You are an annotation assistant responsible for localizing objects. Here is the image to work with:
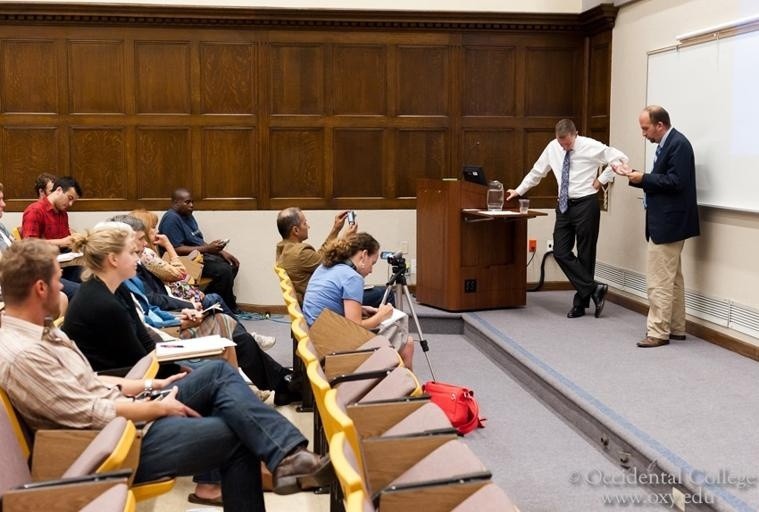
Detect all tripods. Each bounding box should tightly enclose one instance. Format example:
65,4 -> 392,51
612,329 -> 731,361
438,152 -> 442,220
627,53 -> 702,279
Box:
379,267 -> 436,382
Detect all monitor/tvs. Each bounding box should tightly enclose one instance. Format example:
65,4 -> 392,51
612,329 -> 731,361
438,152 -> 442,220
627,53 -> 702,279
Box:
463,165 -> 487,186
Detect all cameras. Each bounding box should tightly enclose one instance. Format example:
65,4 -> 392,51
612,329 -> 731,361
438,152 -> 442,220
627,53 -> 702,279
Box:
380,249 -> 406,267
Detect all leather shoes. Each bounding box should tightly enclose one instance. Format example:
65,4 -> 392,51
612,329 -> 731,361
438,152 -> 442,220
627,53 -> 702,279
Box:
272,446 -> 336,494
637,337 -> 669,347
670,334 -> 686,340
592,283 -> 607,319
568,306 -> 585,318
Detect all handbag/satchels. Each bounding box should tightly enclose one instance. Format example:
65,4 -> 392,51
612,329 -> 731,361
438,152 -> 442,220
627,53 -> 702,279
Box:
170,280 -> 204,300
423,381 -> 473,427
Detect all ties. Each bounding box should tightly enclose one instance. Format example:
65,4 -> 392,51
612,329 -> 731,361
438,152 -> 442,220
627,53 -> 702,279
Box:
558,150 -> 572,213
643,145 -> 660,208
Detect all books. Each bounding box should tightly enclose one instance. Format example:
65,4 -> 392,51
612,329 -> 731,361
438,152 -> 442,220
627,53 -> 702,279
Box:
374,304 -> 407,337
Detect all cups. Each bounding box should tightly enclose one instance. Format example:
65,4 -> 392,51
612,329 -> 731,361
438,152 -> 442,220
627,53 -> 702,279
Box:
519,199 -> 530,213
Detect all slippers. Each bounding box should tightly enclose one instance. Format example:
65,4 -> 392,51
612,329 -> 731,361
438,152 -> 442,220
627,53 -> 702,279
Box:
189,492 -> 223,507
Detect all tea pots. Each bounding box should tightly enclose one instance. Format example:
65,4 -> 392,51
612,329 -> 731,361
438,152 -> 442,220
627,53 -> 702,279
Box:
486,180 -> 505,211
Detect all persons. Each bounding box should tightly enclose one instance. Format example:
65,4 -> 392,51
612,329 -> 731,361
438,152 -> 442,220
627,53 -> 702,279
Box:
273,205 -> 360,294
301,231 -> 414,373
0,171 -> 335,511
505,116 -> 630,319
609,103 -> 702,349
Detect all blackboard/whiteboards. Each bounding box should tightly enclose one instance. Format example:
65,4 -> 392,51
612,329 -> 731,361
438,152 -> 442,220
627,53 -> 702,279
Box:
643,22 -> 759,214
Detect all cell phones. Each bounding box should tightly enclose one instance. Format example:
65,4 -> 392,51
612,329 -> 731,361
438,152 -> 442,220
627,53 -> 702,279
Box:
221,239 -> 229,244
347,211 -> 355,225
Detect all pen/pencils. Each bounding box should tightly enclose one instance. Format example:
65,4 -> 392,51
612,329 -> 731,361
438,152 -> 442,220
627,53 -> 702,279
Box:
160,345 -> 184,348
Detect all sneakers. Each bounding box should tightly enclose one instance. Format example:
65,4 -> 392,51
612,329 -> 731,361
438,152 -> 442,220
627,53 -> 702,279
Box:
251,332 -> 276,350
255,368 -> 299,406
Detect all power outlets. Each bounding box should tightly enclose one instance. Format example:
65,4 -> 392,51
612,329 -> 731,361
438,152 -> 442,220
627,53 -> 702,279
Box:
529,240 -> 536,252
